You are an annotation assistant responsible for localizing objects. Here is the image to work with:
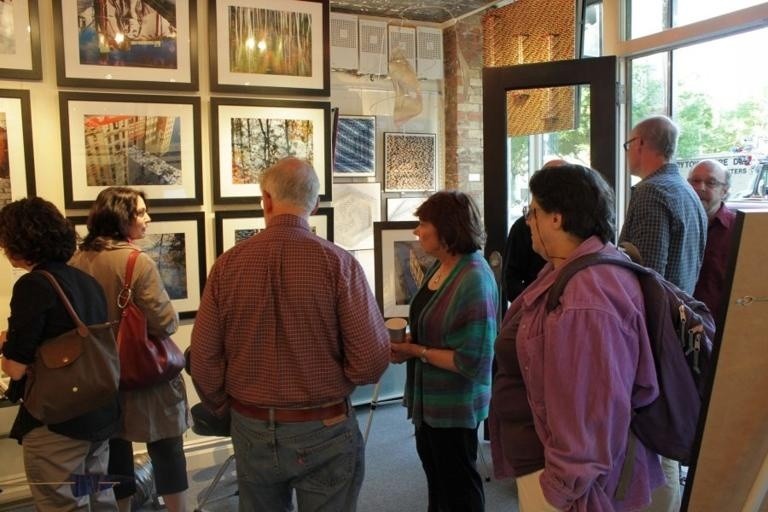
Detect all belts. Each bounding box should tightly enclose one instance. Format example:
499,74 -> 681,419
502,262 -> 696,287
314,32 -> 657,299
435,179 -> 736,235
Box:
229,397 -> 350,423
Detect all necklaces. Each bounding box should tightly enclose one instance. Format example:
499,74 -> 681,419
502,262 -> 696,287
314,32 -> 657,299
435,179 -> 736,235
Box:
433,273 -> 441,284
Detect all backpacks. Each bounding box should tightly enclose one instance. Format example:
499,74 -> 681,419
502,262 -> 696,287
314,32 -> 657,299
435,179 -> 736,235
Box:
546,241 -> 717,466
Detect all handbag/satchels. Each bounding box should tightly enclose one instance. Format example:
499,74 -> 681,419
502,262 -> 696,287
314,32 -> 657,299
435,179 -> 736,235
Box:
21,270 -> 122,424
115,249 -> 186,390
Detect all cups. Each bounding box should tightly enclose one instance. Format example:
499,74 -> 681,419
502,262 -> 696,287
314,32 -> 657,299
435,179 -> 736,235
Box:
384,318 -> 407,344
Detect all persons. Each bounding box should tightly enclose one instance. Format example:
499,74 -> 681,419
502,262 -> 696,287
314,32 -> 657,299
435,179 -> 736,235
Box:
685,158 -> 738,322
189,155 -> 393,512
501,160 -> 572,306
486,164 -> 683,512
0,195 -> 118,512
617,115 -> 709,512
390,189 -> 500,511
66,186 -> 196,512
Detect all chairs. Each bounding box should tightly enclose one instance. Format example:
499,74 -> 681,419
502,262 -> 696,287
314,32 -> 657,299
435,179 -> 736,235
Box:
184,344 -> 237,512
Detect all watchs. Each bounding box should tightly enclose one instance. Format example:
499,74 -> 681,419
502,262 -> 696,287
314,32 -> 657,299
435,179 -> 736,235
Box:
420,345 -> 430,364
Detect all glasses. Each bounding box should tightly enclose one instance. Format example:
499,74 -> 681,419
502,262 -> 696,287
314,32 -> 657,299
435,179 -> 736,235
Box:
623,136 -> 638,151
522,206 -> 542,219
687,176 -> 727,188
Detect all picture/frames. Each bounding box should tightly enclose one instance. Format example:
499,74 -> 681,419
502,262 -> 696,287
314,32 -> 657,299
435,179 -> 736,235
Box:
66,211 -> 208,321
214,207 -> 335,260
383,132 -> 436,193
374,220 -> 438,326
386,197 -> 431,221
0,0 -> 44,81
209,96 -> 332,205
332,114 -> 379,184
0,89 -> 36,209
52,0 -> 199,92
58,91 -> 204,211
332,182 -> 381,251
208,0 -> 330,97
